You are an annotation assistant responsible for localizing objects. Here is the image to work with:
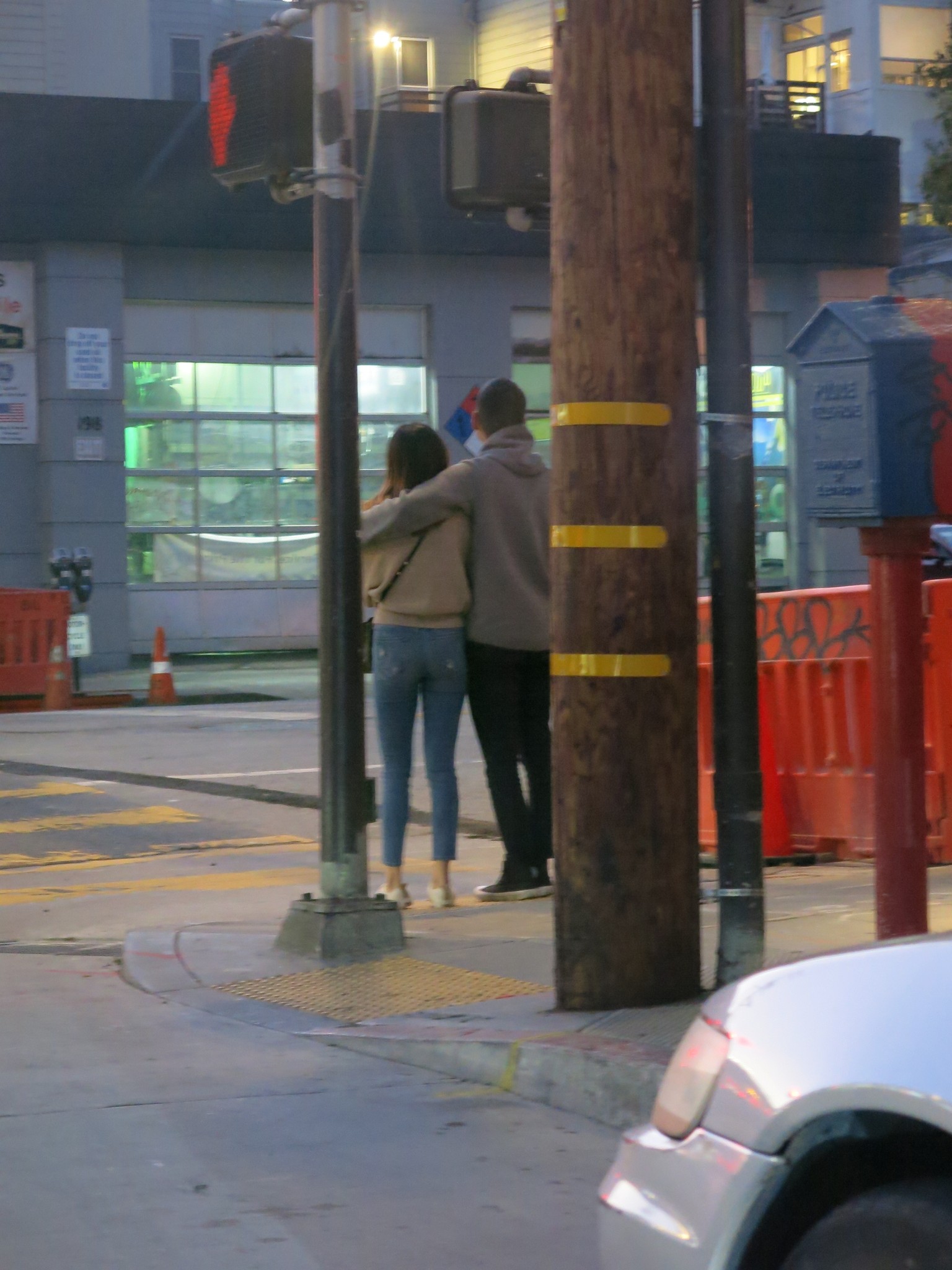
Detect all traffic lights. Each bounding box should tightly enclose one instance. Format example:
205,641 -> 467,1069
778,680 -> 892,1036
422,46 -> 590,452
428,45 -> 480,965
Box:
207,27 -> 314,185
752,128 -> 911,269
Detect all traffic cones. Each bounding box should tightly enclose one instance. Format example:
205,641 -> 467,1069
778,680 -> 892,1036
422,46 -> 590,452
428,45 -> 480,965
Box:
146,627 -> 184,707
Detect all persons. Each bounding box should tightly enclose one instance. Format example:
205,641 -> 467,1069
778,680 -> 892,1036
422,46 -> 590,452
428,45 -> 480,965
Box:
359,378 -> 554,900
360,423 -> 474,908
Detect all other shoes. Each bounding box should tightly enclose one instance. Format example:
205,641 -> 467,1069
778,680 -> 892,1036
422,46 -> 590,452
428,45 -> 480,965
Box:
428,885 -> 454,908
377,882 -> 412,911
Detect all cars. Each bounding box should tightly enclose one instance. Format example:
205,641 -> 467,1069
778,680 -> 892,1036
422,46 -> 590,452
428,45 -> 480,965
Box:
596,930 -> 952,1270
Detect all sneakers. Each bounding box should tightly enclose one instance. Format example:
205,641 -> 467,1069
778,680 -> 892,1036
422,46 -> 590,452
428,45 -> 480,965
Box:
473,856 -> 540,903
531,859 -> 554,896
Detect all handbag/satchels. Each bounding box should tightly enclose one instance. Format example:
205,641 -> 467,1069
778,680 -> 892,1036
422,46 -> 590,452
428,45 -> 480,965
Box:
362,616 -> 374,674
